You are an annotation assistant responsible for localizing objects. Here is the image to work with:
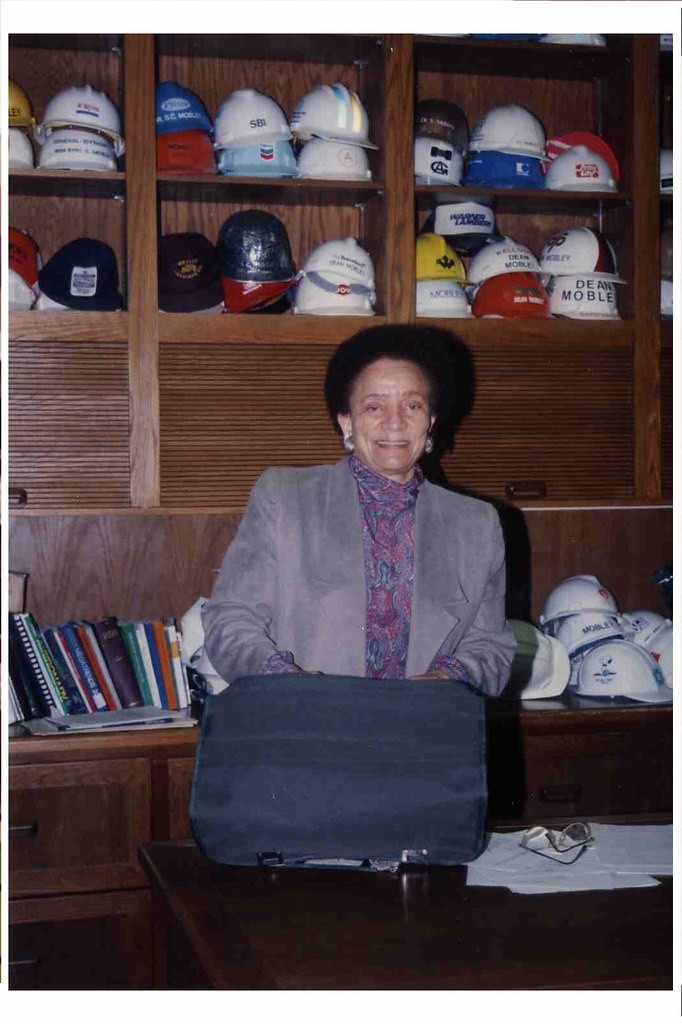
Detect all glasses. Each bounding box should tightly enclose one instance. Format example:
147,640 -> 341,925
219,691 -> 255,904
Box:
519,820 -> 597,867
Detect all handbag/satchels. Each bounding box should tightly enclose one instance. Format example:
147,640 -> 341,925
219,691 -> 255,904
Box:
186,672 -> 490,863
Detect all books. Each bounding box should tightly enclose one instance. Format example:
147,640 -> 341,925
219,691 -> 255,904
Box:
9,612 -> 192,725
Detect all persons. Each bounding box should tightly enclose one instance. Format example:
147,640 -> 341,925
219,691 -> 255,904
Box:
196,321 -> 517,697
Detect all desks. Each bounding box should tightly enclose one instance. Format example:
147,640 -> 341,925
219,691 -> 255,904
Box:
136,812 -> 672,989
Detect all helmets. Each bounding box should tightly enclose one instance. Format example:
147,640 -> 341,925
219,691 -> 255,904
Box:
8,126 -> 38,168
415,204 -> 625,325
469,107 -> 557,165
291,82 -> 377,146
158,126 -> 215,176
416,96 -> 467,185
219,210 -> 381,317
9,78 -> 41,127
219,144 -> 293,173
658,145 -> 672,329
297,138 -> 376,187
546,123 -> 617,193
38,129 -> 119,173
534,572 -> 682,702
154,77 -> 213,133
467,151 -> 548,188
34,85 -> 130,157
207,90 -> 293,146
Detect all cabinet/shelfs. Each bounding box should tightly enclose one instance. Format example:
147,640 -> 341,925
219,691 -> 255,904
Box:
9,508 -> 673,990
10,34 -> 673,511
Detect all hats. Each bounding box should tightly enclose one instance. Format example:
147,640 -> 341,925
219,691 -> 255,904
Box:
9,226 -> 44,314
157,229 -> 220,312
41,234 -> 121,310
